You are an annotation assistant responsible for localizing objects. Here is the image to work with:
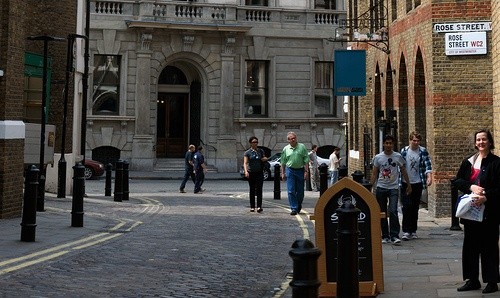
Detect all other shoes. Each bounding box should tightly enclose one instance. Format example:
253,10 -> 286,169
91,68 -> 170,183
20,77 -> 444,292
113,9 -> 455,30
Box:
456,280 -> 481,292
482,284 -> 499,294
382,237 -> 390,244
200,189 -> 206,193
257,208 -> 263,213
390,237 -> 401,244
250,208 -> 255,212
401,233 -> 418,240
290,206 -> 302,215
179,189 -> 187,193
194,191 -> 203,194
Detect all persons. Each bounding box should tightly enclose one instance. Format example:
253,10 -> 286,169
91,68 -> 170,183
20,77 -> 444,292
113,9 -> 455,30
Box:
397,131 -> 433,240
179,145 -> 206,193
244,137 -> 269,212
193,146 -> 208,194
309,145 -> 322,193
328,148 -> 343,186
455,130 -> 500,294
281,132 -> 310,216
370,135 -> 412,244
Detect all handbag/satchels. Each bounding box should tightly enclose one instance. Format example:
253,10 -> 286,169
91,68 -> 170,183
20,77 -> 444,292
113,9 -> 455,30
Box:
455,194 -> 485,223
261,160 -> 270,170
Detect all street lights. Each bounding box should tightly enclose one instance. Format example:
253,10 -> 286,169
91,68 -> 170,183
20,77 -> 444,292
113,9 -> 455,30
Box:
27,34 -> 67,213
57,32 -> 88,198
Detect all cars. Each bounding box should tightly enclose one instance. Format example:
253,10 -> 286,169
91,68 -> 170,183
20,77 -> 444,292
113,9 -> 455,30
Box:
240,152 -> 331,182
81,158 -> 104,181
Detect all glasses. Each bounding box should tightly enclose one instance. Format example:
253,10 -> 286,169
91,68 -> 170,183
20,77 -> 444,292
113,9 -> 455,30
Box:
251,142 -> 257,143
